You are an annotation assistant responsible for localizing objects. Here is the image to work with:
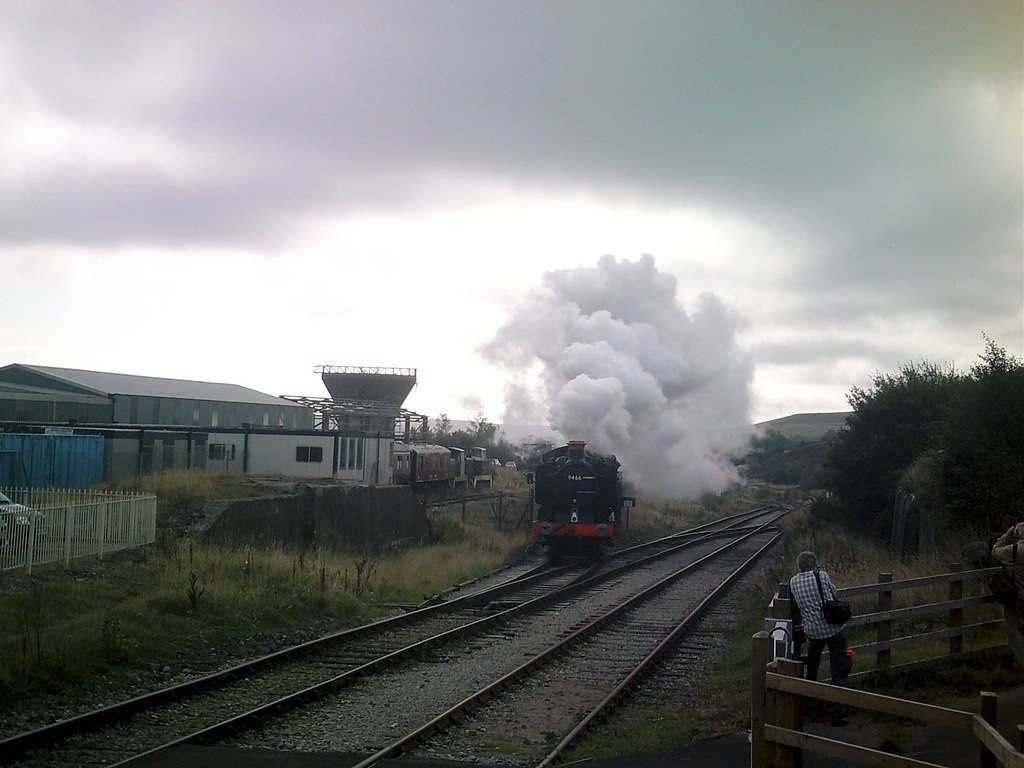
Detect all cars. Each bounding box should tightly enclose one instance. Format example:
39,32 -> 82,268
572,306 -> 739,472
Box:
0,491 -> 47,556
490,458 -> 502,466
505,461 -> 516,469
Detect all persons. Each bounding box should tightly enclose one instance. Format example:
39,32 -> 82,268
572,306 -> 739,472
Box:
790,551 -> 854,729
991,521 -> 1024,674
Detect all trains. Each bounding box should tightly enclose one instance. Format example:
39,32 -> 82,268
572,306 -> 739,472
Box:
389,440 -> 452,486
532,440 -> 635,561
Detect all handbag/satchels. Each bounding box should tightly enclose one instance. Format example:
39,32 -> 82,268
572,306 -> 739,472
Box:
823,600 -> 851,625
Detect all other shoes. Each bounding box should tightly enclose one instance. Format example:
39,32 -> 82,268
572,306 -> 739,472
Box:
832,713 -> 850,727
